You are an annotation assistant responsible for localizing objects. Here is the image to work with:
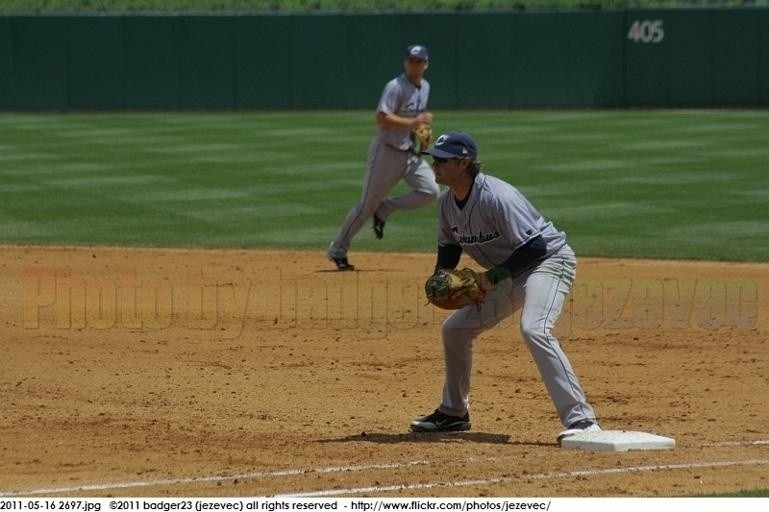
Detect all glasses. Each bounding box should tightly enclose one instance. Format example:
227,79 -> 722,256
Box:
433,156 -> 449,164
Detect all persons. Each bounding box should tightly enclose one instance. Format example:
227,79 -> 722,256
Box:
326,45 -> 442,271
409,131 -> 602,442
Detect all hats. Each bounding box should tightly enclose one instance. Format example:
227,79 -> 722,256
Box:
419,132 -> 478,159
403,44 -> 429,61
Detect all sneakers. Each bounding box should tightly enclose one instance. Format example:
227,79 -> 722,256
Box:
556,417 -> 600,443
372,213 -> 385,239
326,252 -> 354,270
410,409 -> 471,433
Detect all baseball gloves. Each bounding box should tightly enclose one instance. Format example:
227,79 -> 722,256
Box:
412,121 -> 433,156
425,268 -> 488,309
